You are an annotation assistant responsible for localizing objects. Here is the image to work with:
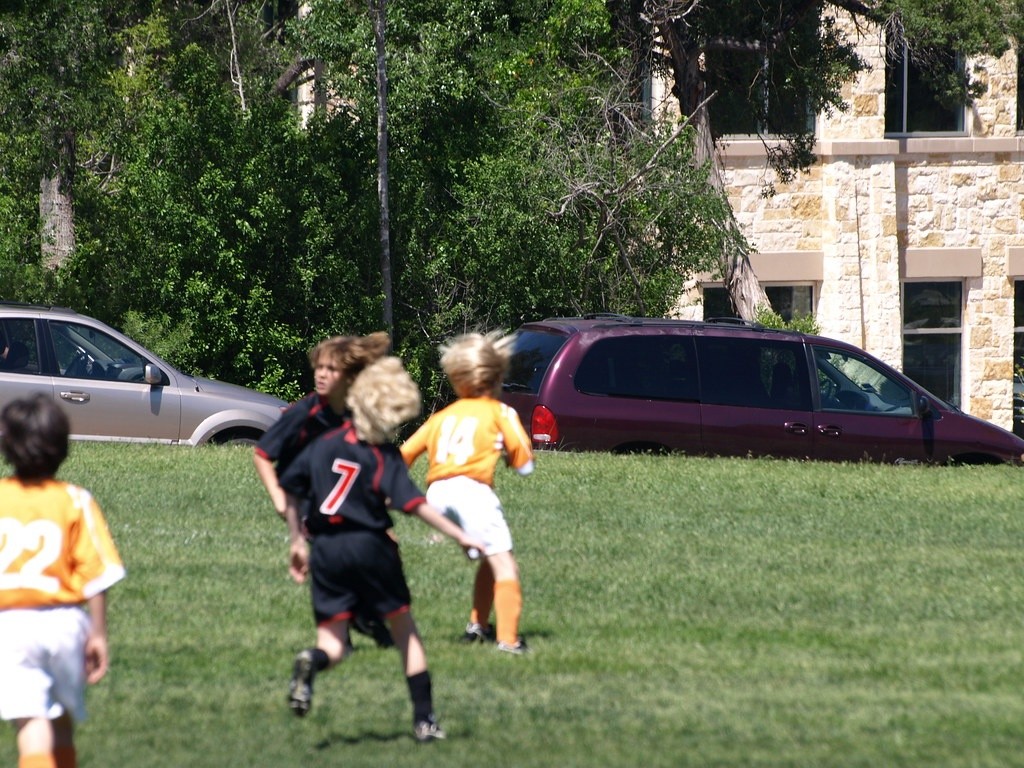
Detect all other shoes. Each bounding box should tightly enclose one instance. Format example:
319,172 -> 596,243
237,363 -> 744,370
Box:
498,640 -> 525,654
465,621 -> 495,639
287,651 -> 318,718
413,716 -> 447,741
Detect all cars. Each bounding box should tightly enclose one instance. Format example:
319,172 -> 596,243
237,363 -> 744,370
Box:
0,304 -> 291,448
498,312 -> 1023,467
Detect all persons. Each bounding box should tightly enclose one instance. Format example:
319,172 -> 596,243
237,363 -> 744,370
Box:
0,394 -> 127,768
253,334 -> 485,742
398,333 -> 534,654
